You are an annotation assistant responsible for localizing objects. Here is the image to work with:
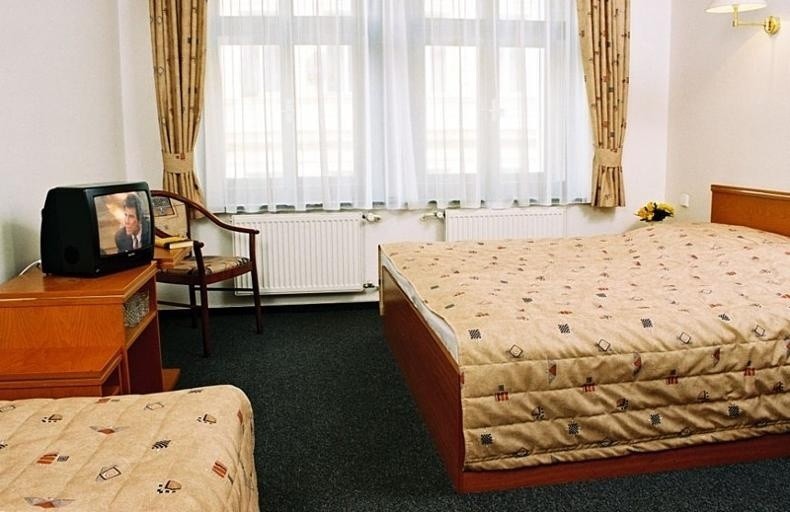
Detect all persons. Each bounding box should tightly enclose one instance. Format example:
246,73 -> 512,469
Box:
114,193 -> 150,253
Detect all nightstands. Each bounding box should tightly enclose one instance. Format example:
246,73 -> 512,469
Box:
0,343 -> 128,402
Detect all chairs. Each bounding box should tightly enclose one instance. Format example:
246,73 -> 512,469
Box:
150,190 -> 266,360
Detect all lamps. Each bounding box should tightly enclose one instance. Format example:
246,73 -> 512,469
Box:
705,1 -> 781,37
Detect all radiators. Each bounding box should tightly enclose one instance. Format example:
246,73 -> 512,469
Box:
422,206 -> 568,243
231,211 -> 380,296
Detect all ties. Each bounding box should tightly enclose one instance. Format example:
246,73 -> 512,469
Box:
134,237 -> 138,249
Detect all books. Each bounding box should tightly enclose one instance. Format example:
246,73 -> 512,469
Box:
153,237 -> 194,250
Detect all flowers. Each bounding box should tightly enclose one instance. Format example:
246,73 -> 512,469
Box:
634,202 -> 677,224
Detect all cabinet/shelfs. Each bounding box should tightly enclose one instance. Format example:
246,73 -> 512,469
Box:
1,258 -> 181,403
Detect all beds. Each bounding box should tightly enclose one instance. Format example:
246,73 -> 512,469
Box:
376,184 -> 790,494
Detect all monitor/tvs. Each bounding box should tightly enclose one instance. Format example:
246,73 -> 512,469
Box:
40,182 -> 155,278
